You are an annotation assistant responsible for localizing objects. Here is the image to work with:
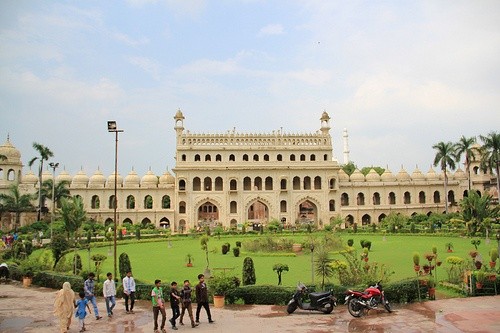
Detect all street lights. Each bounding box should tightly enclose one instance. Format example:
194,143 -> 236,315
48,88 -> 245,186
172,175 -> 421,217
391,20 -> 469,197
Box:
49,162 -> 60,242
107,121 -> 124,287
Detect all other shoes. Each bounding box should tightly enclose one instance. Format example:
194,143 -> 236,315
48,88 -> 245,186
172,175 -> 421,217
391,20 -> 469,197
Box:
160,327 -> 166,333
179,322 -> 183,325
96,317 -> 101,320
192,324 -> 196,328
130,309 -> 135,314
67,326 -> 70,330
195,322 -> 199,326
108,315 -> 112,318
209,319 -> 214,322
172,327 -> 178,330
154,330 -> 159,333
126,311 -> 131,314
110,310 -> 113,315
80,327 -> 86,333
170,319 -> 176,324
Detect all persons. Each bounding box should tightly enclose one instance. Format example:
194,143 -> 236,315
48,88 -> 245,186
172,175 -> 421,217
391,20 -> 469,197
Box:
179,280 -> 199,328
151,279 -> 167,333
84,272 -> 103,320
73,292 -> 91,332
169,281 -> 181,330
122,270 -> 135,314
1,231 -> 18,245
52,282 -> 76,333
103,272 -> 116,317
195,274 -> 215,323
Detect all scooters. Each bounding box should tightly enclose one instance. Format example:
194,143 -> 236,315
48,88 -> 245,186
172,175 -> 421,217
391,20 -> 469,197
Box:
286,281 -> 338,314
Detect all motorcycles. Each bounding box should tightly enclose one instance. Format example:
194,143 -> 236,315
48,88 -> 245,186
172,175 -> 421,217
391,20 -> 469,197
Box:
344,280 -> 393,317
